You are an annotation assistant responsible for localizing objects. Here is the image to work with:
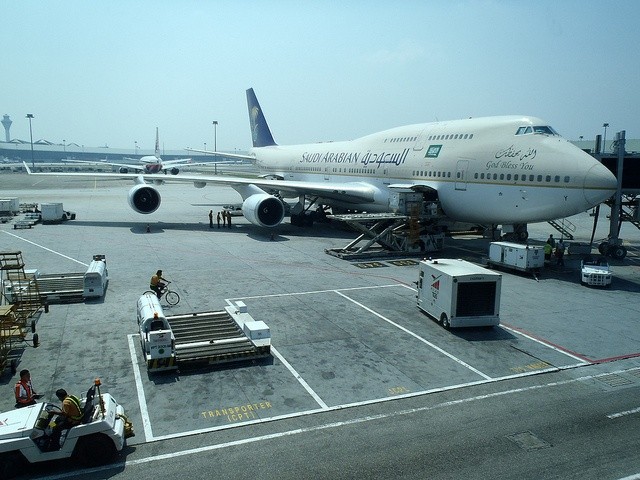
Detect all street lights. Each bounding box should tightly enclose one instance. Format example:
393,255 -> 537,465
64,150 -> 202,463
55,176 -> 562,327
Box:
212,121 -> 218,174
602,123 -> 609,153
25,114 -> 35,169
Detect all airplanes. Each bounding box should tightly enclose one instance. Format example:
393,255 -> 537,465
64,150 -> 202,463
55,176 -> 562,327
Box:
103,156 -> 191,175
22,87 -> 619,242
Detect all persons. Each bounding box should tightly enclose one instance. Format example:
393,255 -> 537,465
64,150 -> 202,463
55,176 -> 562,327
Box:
270,231 -> 274,241
227,212 -> 232,227
43,388 -> 84,451
216,212 -> 222,228
542,240 -> 553,261
14,369 -> 36,408
547,233 -> 555,248
556,239 -> 566,267
575,256 -> 584,283
208,209 -> 214,229
222,210 -> 227,228
149,269 -> 171,299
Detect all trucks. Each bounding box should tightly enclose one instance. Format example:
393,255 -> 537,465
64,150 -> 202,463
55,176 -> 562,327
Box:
415,257 -> 502,330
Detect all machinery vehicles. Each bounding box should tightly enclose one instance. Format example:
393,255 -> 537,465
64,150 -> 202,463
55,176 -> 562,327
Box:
581,257 -> 613,288
0,378 -> 134,480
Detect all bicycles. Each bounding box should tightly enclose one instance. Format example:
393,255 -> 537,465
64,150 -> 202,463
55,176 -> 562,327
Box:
142,281 -> 180,305
